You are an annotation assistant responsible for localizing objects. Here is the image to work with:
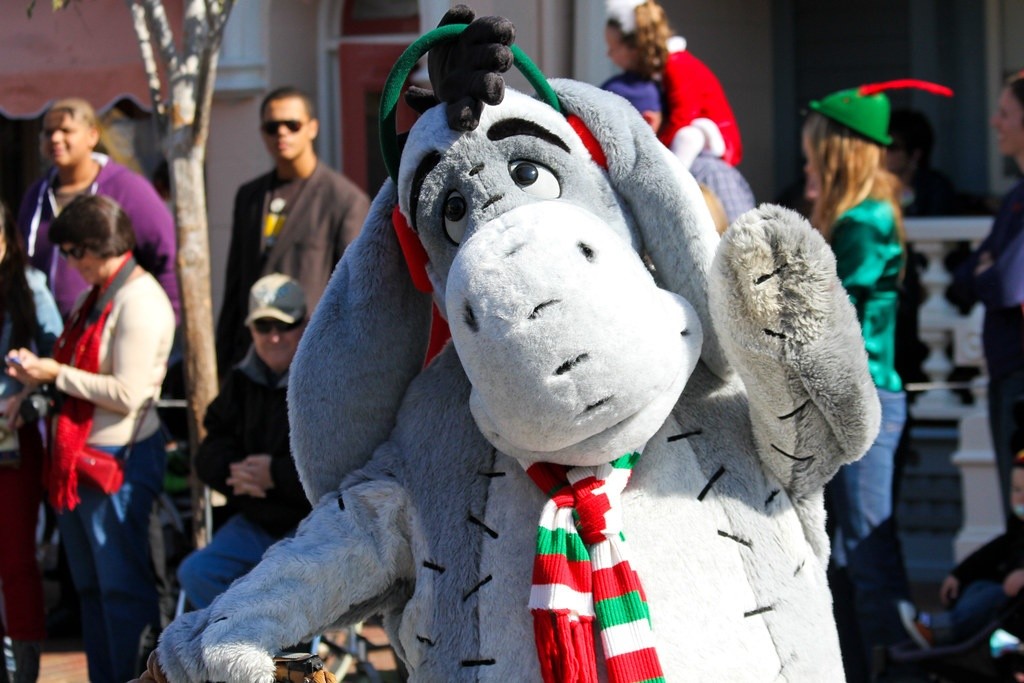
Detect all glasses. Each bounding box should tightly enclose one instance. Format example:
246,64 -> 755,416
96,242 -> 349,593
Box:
58,243 -> 89,261
253,319 -> 306,332
260,119 -> 312,136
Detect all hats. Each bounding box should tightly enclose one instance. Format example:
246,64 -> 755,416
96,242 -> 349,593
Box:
808,78 -> 956,145
242,272 -> 308,329
601,71 -> 663,113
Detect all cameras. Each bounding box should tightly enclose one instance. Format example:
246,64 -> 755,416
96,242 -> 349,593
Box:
19,380 -> 69,423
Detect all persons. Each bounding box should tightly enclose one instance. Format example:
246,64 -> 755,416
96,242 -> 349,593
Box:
16,98 -> 182,333
4,195 -> 175,683
800,87 -> 921,683
176,272 -> 320,657
215,86 -> 373,392
895,451 -> 1024,650
603,82 -> 756,233
0,198 -> 65,683
884,108 -> 971,402
603,0 -> 744,167
958,72 -> 1024,529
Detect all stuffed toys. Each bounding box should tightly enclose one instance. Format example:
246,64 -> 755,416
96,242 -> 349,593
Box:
156,4 -> 882,683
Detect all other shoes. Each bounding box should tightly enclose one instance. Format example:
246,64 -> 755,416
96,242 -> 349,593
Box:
897,599 -> 935,651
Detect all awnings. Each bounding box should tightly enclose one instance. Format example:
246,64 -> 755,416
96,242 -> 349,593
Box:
0,0 -> 184,120
